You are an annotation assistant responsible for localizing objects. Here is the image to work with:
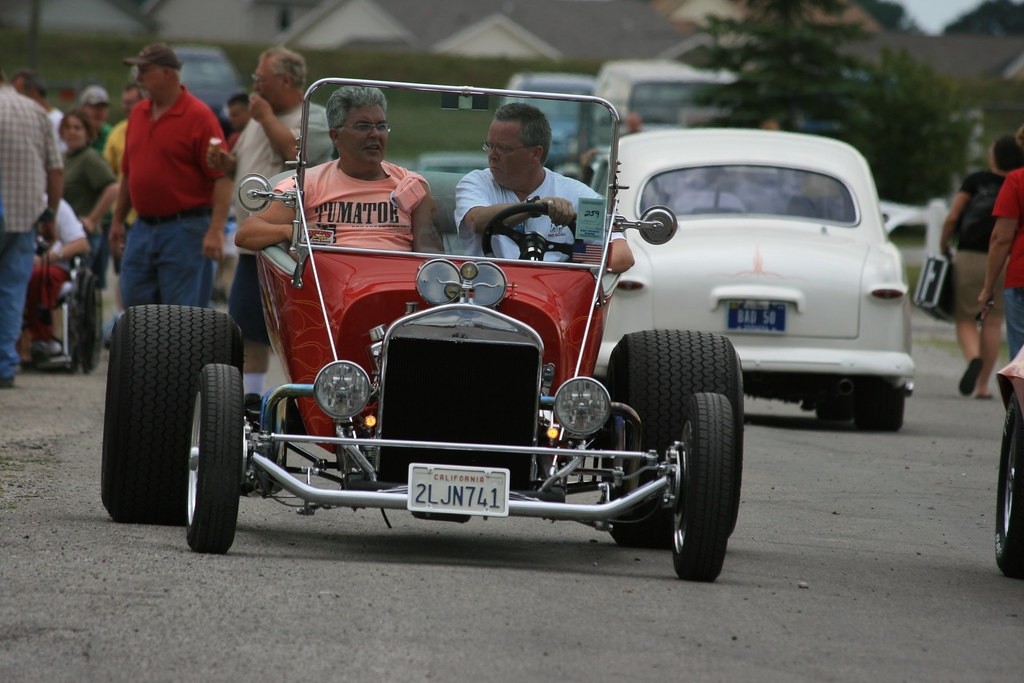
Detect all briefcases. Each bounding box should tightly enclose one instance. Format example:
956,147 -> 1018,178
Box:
913,250 -> 955,322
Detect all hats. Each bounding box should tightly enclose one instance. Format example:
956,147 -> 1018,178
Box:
80,85 -> 109,105
123,43 -> 181,70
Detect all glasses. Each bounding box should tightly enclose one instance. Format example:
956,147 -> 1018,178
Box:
336,123 -> 391,134
250,74 -> 284,83
481,142 -> 537,154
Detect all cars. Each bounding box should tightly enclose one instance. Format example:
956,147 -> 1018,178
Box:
879,199 -> 949,266
577,125 -> 916,432
125,45 -> 245,139
417,152 -> 493,176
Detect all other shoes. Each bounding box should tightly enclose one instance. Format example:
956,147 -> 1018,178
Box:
959,359 -> 983,397
32,344 -> 48,363
976,396 -> 991,399
0,376 -> 14,387
104,312 -> 119,343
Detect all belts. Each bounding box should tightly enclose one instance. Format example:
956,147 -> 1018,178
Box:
139,207 -> 212,225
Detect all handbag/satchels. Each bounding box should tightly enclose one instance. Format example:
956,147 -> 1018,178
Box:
37,304 -> 51,325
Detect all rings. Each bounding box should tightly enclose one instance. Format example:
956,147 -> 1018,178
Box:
548,199 -> 554,206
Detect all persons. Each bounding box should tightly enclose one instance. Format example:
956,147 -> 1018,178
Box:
939,121 -> 1024,443
234,84 -> 446,255
0,31 -> 257,388
108,43 -> 233,311
454,103 -> 635,274
189,46 -> 338,473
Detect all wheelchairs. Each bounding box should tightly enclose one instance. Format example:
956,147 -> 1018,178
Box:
21,247 -> 103,375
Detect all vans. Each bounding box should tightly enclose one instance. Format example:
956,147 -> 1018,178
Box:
592,56 -> 742,136
499,71 -> 597,168
